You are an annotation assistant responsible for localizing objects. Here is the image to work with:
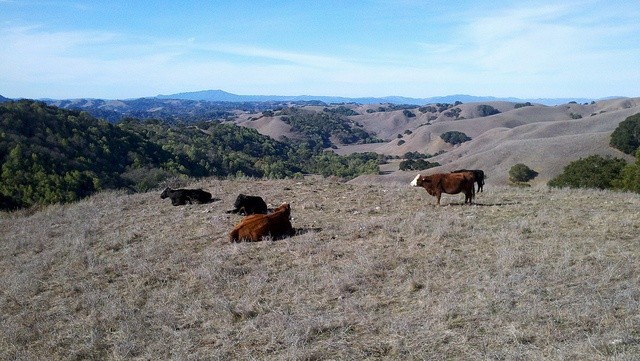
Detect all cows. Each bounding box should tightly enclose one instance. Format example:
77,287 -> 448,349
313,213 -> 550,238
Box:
161,187 -> 213,206
225,193 -> 297,242
409,169 -> 485,205
